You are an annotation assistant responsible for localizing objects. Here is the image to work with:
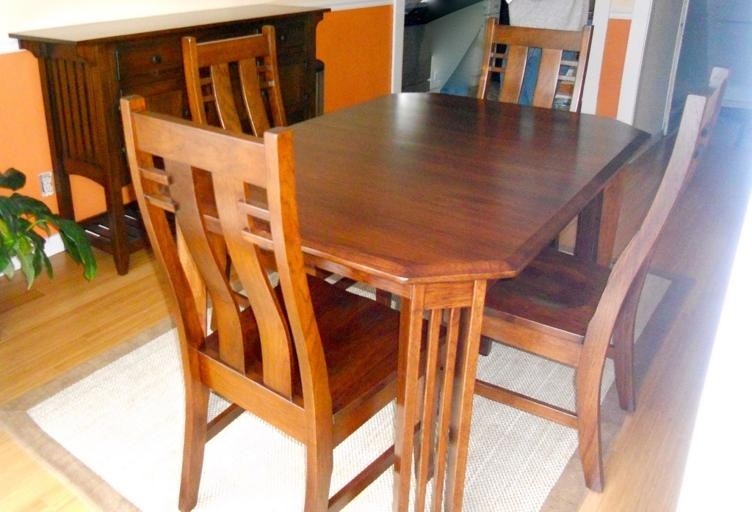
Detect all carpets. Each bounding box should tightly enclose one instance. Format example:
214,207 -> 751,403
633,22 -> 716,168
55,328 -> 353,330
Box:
2,258 -> 695,512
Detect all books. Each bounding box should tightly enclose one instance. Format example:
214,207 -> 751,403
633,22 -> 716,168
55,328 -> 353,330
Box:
553,69 -> 577,111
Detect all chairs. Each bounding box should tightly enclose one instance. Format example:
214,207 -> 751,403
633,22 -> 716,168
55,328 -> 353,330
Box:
118,91 -> 451,512
178,25 -> 393,334
373,16 -> 598,361
437,64 -> 732,493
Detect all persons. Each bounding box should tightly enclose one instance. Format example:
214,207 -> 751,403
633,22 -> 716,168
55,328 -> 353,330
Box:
500,0 -> 590,106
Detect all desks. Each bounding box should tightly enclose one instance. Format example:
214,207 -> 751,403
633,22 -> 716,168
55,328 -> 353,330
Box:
159,91 -> 656,485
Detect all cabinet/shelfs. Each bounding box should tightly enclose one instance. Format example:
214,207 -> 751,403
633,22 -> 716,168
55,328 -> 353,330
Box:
9,5 -> 336,276
